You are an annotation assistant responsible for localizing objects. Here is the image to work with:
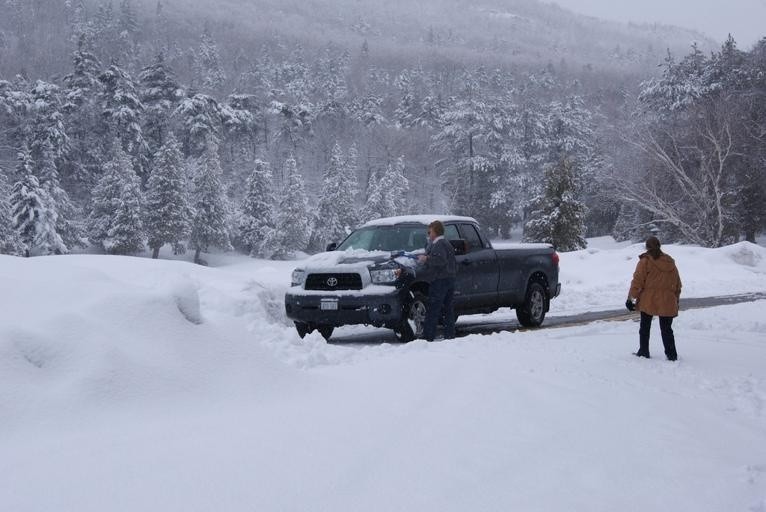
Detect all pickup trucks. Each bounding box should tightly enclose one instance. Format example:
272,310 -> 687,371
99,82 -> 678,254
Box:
283,211 -> 562,345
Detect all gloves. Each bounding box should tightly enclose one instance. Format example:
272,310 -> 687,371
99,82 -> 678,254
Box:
625,300 -> 636,311
416,255 -> 428,266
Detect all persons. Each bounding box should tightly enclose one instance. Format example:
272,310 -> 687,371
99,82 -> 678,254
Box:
624,236 -> 682,361
417,220 -> 459,341
